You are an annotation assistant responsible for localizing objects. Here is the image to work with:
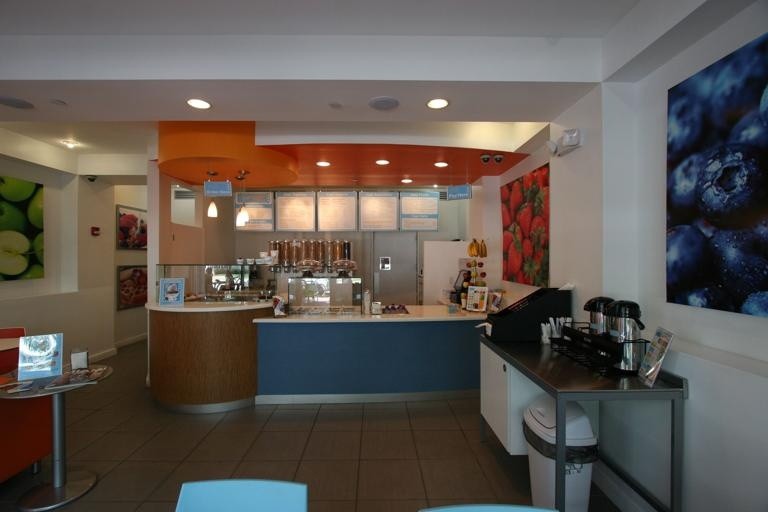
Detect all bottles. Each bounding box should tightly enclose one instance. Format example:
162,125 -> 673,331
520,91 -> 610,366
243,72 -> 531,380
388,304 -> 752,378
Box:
270,238 -> 352,266
583,296 -> 643,372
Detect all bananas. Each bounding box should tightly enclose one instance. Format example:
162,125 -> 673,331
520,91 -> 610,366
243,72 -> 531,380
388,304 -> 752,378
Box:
466,238 -> 487,258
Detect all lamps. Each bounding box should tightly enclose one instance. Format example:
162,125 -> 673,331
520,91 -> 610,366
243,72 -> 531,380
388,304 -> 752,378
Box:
206,171 -> 218,218
235,170 -> 251,226
235,176 -> 250,222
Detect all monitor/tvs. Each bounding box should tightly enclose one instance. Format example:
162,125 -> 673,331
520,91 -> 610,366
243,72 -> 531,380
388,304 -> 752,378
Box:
453,269 -> 471,291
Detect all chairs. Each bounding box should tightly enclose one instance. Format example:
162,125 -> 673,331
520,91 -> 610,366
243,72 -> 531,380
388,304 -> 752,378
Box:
1,327 -> 53,486
174,479 -> 309,512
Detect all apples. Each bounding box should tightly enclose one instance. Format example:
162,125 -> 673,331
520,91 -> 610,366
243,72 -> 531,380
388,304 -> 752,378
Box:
0,175 -> 44,280
460,262 -> 486,298
399,304 -> 405,309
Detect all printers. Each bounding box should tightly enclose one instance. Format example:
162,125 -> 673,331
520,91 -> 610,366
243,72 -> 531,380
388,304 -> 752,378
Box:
483,287 -> 572,343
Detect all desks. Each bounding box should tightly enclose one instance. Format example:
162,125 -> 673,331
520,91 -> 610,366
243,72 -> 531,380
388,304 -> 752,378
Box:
479,334 -> 688,512
0,365 -> 113,511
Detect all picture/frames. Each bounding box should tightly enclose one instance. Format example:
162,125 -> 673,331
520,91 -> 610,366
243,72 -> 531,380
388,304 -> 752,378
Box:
117,265 -> 147,310
159,277 -> 184,307
116,204 -> 148,250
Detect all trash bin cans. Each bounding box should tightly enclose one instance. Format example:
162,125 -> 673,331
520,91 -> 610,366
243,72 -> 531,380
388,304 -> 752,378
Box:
523,393 -> 598,512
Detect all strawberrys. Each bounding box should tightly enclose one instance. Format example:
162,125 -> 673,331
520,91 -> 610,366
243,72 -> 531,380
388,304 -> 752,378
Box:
500,168 -> 549,287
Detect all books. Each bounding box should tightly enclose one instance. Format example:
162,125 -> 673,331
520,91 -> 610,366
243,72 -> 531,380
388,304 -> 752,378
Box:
45,367 -> 108,390
0,380 -> 40,395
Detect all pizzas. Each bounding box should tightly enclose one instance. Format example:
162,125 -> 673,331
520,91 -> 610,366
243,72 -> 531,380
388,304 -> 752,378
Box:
118,279 -> 147,305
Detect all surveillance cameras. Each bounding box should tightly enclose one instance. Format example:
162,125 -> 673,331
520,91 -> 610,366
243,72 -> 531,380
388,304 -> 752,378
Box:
493,155 -> 504,164
480,154 -> 492,165
